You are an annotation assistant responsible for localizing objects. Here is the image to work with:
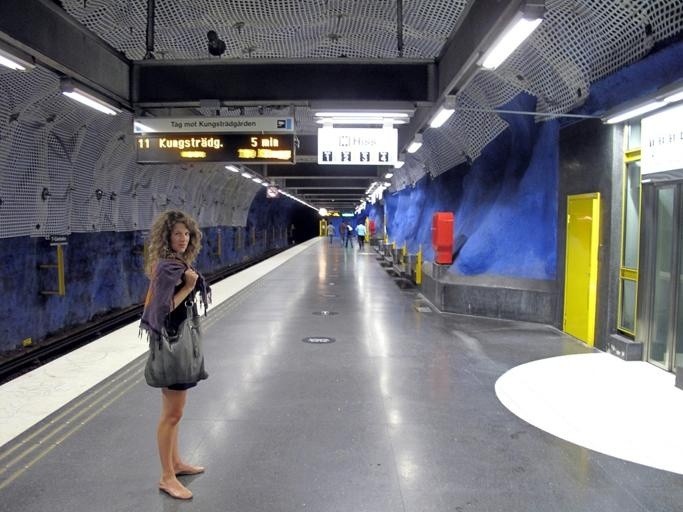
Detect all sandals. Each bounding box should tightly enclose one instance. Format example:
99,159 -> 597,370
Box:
175,465 -> 205,476
158,483 -> 193,500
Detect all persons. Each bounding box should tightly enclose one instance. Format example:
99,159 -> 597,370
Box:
138,209 -> 211,501
326,221 -> 368,250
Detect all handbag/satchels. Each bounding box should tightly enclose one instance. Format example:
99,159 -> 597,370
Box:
144,300 -> 210,388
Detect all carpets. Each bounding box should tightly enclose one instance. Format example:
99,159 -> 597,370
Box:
492,351 -> 683,476
